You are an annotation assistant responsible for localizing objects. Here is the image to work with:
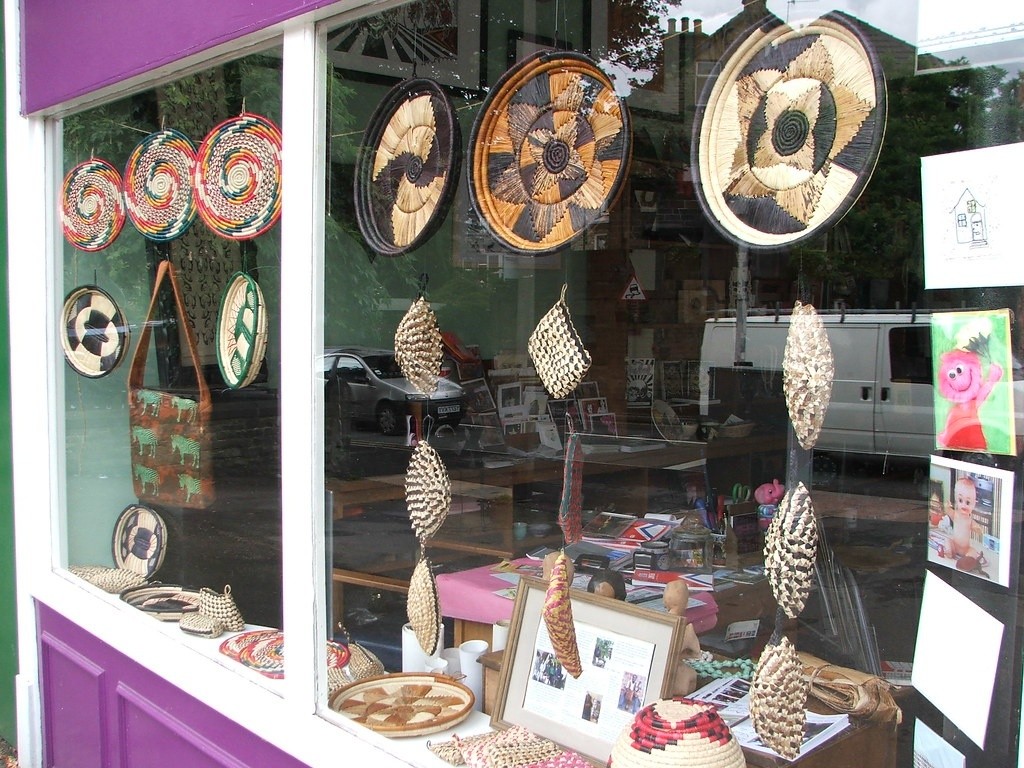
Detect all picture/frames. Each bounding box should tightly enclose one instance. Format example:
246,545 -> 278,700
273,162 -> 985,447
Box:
242,0 -> 488,100
490,575 -> 688,768
452,160 -> 561,269
507,30 -> 572,70
134,61 -> 268,389
583,0 -> 686,123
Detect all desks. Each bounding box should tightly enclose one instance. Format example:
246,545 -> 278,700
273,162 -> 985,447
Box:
435,550 -> 797,658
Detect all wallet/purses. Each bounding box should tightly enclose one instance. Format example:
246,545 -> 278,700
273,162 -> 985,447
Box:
179,612 -> 225,639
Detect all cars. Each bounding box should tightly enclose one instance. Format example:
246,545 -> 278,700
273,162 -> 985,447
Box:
314,348 -> 466,437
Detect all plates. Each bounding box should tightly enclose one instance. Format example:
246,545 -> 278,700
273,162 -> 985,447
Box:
651,399 -> 684,445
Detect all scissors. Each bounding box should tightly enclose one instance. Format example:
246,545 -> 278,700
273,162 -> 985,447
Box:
732,484 -> 751,503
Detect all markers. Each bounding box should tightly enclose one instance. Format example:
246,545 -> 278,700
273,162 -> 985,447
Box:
695,494 -> 724,531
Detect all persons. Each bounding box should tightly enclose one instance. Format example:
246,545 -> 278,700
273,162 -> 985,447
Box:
603,648 -> 612,669
625,674 -> 641,711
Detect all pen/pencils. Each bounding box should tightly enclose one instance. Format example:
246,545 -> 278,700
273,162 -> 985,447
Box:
730,715 -> 750,729
629,593 -> 663,603
723,513 -> 728,530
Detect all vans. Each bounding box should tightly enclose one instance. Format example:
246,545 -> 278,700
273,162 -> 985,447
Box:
697,314 -> 1024,474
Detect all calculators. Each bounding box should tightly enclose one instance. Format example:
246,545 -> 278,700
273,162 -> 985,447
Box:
573,554 -> 610,574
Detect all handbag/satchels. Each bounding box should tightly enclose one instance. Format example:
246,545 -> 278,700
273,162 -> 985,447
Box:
127,261 -> 217,511
197,585 -> 245,633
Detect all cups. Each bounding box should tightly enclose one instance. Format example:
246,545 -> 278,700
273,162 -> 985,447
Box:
700,422 -> 720,443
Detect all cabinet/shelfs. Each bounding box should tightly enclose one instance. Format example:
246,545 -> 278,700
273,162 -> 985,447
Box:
476,650 -> 897,768
586,157 -> 870,431
326,431 -> 785,634
